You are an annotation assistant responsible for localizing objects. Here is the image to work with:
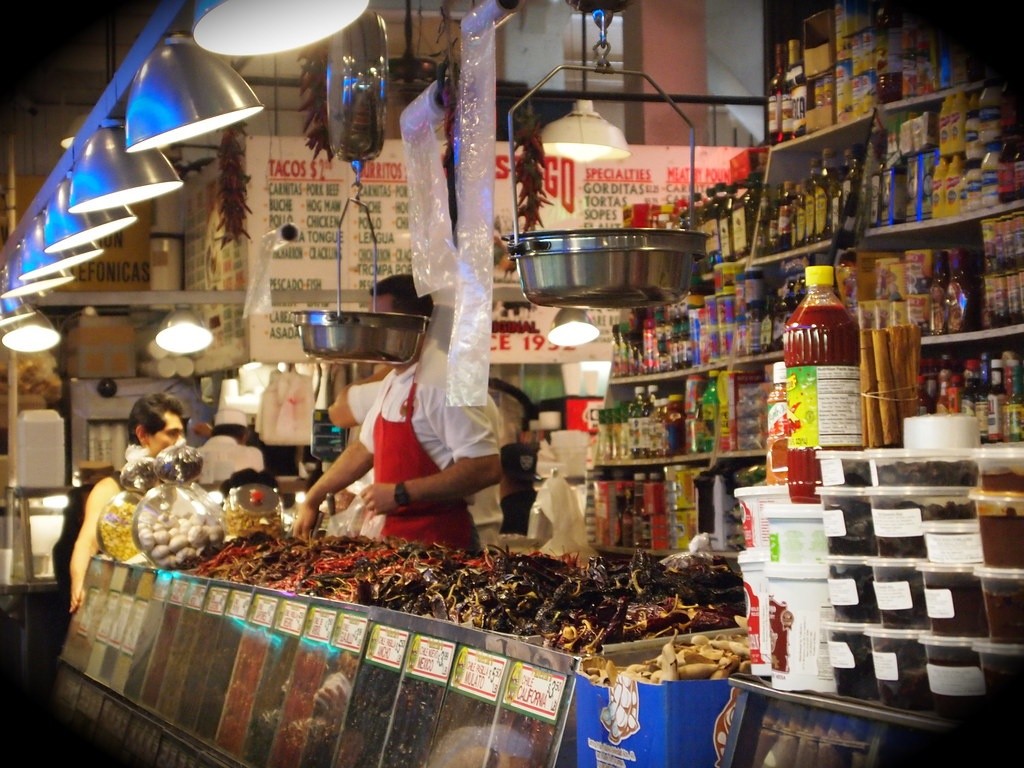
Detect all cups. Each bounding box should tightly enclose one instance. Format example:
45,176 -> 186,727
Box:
137,336 -> 194,378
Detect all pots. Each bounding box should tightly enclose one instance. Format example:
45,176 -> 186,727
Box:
292,310 -> 430,365
501,228 -> 710,306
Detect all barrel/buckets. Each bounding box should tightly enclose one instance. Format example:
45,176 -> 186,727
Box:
732,483 -> 837,695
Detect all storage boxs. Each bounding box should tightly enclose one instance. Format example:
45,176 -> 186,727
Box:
66,326 -> 138,378
3,174 -> 150,291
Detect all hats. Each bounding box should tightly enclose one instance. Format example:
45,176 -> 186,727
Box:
214,378 -> 252,428
548,429 -> 588,479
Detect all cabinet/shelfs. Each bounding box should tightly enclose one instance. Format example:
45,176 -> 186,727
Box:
592,68 -> 1024,562
21,293 -> 250,489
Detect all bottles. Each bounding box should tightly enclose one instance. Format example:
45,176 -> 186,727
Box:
628,369 -> 719,458
664,174 -> 760,263
764,149 -> 860,265
928,248 -> 977,335
635,473 -> 661,548
768,39 -> 806,146
95,484 -> 283,573
782,266 -> 863,504
931,77 -> 1024,221
766,361 -> 789,487
918,351 -> 1024,443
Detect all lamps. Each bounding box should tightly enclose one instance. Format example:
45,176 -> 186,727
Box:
549,306 -> 601,345
0,296 -> 36,327
1,244 -> 76,298
0,306 -> 62,352
541,2 -> 631,158
152,303 -> 214,353
67,125 -> 185,213
19,209 -> 103,280
192,0 -> 372,56
44,179 -> 138,253
126,36 -> 265,152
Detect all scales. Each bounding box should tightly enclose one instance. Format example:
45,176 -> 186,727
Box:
500,0 -> 709,318
310,408 -> 350,517
290,8 -> 433,365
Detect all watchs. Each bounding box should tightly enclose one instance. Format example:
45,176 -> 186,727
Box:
394,481 -> 410,507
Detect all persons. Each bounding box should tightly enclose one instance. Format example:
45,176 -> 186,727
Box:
52,377 -> 265,685
291,273 -> 503,560
499,444 -> 539,537
